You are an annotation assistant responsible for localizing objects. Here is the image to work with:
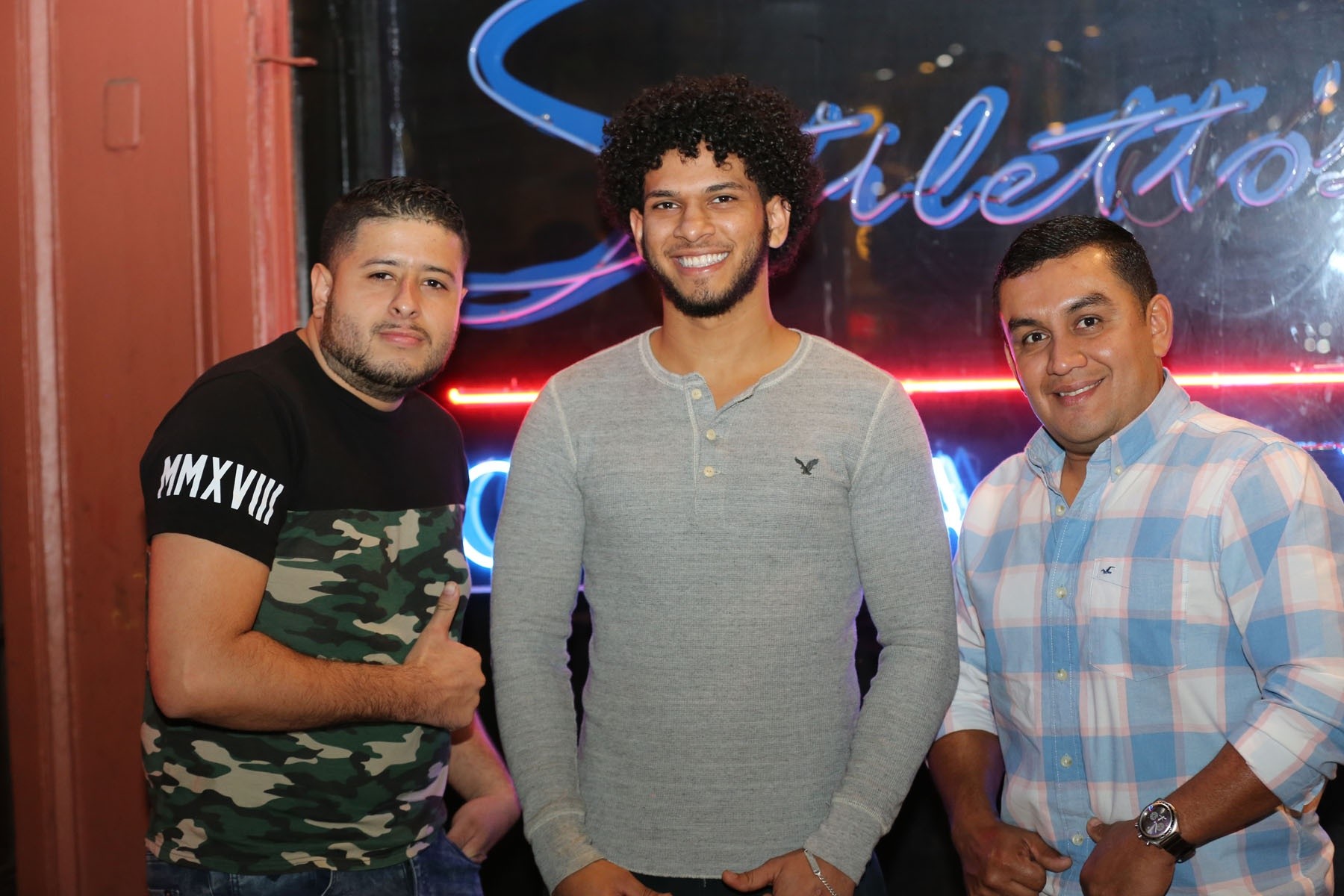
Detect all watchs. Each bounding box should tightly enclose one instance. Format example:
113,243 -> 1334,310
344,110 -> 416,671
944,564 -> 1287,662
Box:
1133,798 -> 1196,864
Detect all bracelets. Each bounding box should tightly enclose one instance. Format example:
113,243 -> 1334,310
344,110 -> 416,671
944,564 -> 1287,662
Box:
804,849 -> 837,896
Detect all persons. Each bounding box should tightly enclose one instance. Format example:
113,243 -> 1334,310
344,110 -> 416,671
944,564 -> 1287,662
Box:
492,73 -> 959,896
923,216 -> 1344,896
137,176 -> 519,896
